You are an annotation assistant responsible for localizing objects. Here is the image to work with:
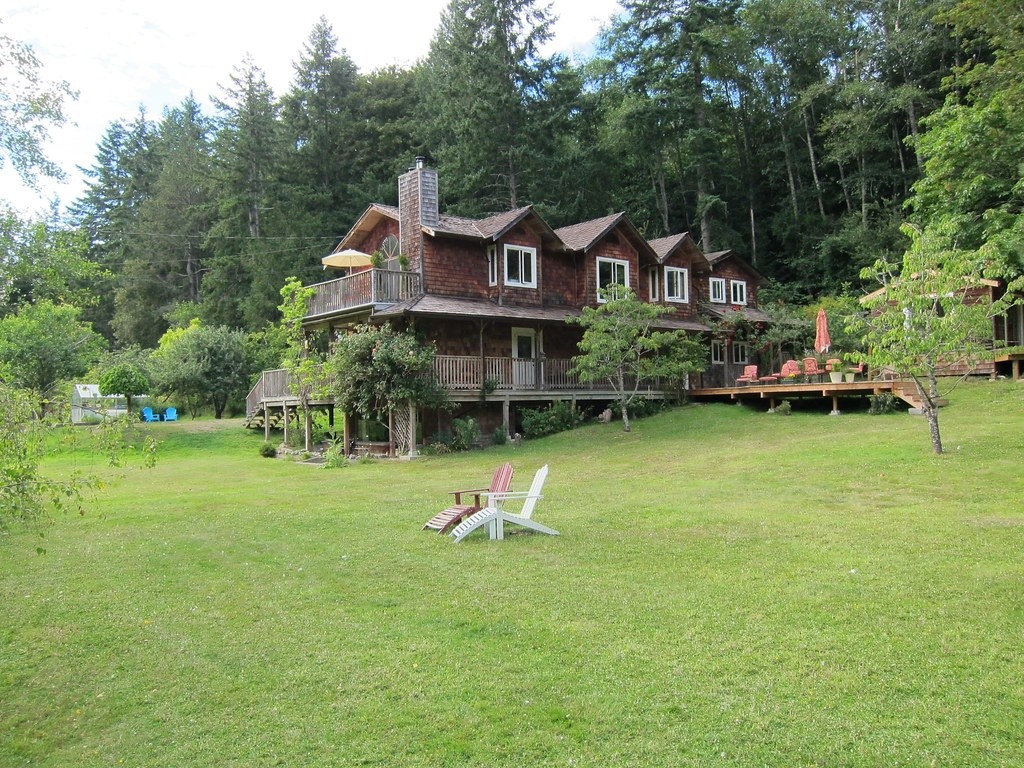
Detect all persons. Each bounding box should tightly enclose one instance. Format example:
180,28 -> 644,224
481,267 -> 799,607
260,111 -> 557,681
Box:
903,304 -> 914,337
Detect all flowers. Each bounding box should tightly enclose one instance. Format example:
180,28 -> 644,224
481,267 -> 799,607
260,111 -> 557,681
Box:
703,304 -> 772,355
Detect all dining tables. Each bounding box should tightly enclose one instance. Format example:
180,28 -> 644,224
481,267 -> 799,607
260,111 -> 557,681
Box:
817,362 -> 831,383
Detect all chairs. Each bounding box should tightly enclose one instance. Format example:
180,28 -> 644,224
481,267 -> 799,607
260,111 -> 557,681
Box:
164,407 -> 178,422
143,407 -> 160,423
449,463 -> 560,543
772,363 -> 789,384
736,365 -> 758,386
420,461 -> 514,536
849,362 -> 864,380
787,360 -> 804,384
802,357 -> 825,383
825,359 -> 841,372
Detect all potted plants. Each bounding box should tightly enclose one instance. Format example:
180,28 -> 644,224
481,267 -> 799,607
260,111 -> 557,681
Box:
845,370 -> 855,384
828,362 -> 843,384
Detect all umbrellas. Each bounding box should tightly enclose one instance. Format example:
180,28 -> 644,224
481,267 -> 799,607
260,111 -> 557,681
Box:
813,306 -> 832,353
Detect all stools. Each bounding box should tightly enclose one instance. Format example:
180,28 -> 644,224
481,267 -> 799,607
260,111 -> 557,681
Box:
759,377 -> 778,385
